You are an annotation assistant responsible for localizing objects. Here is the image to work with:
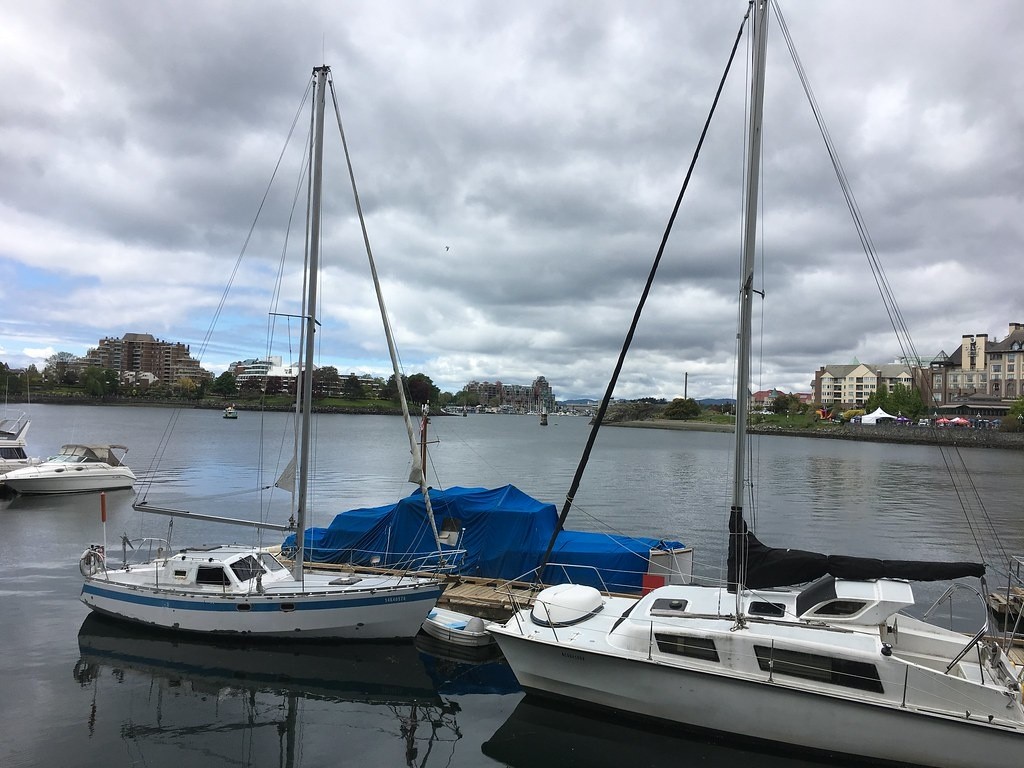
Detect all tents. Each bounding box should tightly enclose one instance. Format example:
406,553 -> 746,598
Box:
850,407 -> 898,426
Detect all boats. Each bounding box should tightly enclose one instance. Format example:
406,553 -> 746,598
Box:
421,605 -> 502,646
0,442 -> 138,496
222,402 -> 238,419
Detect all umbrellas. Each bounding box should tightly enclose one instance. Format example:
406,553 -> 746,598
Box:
894,415 -> 1004,428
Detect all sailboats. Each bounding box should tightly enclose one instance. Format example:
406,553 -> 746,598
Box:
484,1 -> 1024,768
280,394 -> 694,596
77,62 -> 481,647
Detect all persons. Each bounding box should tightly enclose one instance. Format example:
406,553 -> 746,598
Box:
256,572 -> 263,592
815,415 -> 817,422
